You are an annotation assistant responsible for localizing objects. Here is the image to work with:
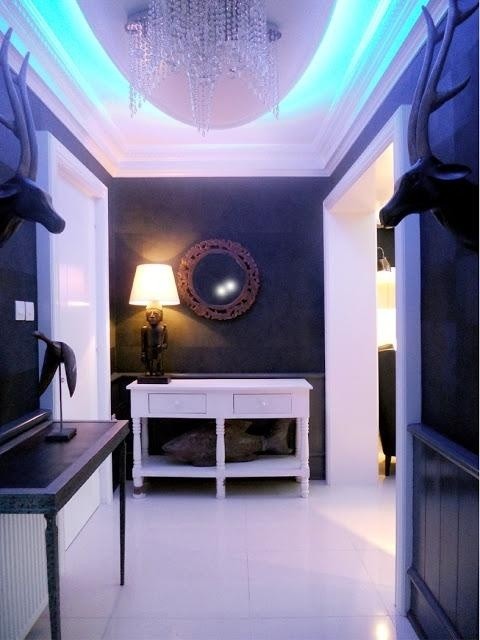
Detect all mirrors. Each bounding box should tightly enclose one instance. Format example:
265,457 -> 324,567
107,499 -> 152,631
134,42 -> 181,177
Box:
177,240 -> 259,322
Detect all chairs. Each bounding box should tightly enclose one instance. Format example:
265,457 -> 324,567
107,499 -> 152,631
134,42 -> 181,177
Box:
378,344 -> 396,478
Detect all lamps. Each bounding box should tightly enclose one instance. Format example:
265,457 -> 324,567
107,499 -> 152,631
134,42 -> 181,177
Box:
125,1 -> 281,138
129,263 -> 181,384
377,247 -> 396,285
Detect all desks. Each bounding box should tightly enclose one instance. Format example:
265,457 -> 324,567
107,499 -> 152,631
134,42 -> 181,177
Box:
0,419 -> 131,640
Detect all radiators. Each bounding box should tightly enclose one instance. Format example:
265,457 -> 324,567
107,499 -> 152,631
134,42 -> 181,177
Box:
0,492 -> 65,640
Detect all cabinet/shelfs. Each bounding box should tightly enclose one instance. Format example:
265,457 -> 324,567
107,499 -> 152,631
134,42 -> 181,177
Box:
125,378 -> 314,500
